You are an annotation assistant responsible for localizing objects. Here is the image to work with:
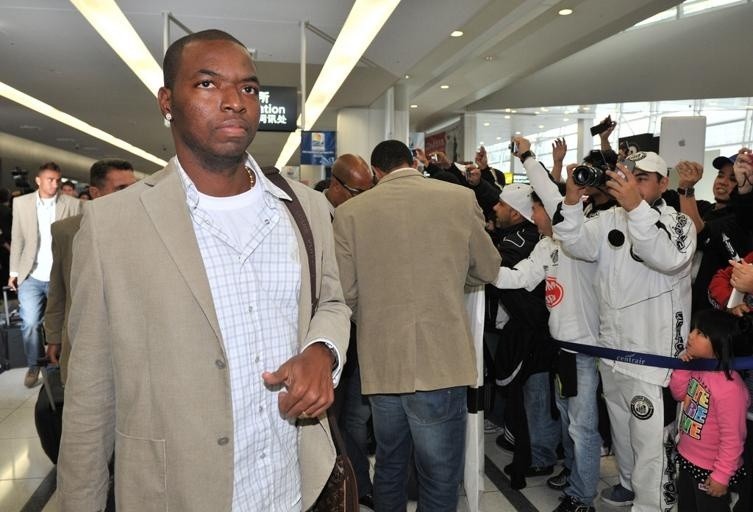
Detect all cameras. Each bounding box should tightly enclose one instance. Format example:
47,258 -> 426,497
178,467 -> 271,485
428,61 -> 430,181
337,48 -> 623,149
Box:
431,153 -> 440,162
508,140 -> 517,153
411,148 -> 417,157
477,144 -> 485,155
572,149 -> 617,188
744,151 -> 753,167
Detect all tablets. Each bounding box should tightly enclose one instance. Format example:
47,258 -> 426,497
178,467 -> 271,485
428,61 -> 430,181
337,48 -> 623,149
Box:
660,116 -> 707,170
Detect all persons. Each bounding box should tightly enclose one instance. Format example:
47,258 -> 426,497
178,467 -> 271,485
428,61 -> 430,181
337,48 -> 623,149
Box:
53,27 -> 355,512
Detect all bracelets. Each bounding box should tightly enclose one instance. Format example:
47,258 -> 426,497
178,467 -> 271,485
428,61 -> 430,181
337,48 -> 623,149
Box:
323,342 -> 337,370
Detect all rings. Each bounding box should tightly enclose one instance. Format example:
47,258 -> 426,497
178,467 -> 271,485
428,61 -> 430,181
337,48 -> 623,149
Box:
302,411 -> 309,418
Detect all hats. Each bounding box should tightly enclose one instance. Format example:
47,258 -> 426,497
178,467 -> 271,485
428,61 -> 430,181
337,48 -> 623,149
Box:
622,151 -> 668,179
712,151 -> 742,170
498,183 -> 540,227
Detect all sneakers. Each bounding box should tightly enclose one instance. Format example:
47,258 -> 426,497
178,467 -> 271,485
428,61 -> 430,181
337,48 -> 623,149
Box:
504,459 -> 554,476
553,495 -> 597,511
602,484 -> 636,507
24,365 -> 40,387
483,418 -> 506,433
358,488 -> 379,511
546,470 -> 575,490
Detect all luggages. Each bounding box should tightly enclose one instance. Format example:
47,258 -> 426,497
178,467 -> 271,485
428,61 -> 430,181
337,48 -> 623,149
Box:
0,286 -> 46,367
34,362 -> 65,466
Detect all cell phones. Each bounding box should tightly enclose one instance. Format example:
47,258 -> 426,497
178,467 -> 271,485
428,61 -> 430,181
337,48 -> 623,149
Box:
607,159 -> 635,193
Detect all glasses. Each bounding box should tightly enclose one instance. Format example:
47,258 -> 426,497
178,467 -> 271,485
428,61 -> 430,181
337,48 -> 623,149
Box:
330,174 -> 365,198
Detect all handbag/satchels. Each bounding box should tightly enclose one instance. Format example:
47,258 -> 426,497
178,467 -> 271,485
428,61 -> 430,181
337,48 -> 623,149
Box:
310,456 -> 361,512
